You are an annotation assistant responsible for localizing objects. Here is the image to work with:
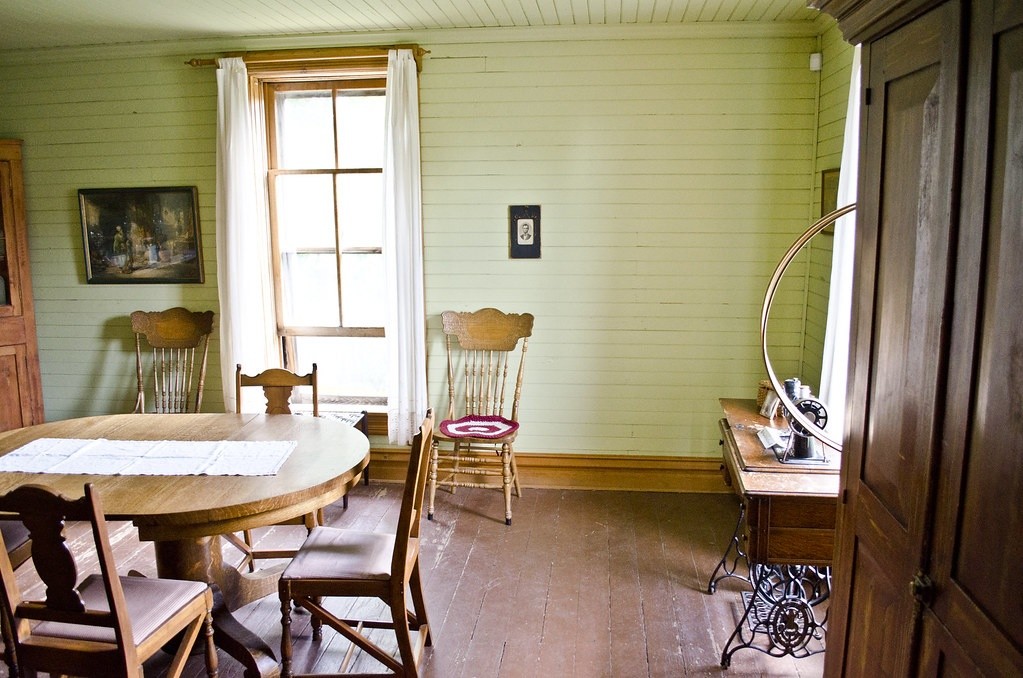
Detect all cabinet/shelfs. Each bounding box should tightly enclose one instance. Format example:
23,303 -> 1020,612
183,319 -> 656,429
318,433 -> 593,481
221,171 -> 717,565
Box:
809,0 -> 1023,678
0,137 -> 46,431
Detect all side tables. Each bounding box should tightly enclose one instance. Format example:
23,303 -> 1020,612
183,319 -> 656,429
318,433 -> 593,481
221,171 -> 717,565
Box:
299,411 -> 371,509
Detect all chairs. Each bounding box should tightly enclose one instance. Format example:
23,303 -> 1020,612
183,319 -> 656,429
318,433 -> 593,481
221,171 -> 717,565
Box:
0,481 -> 221,678
130,307 -> 217,414
278,408 -> 436,678
428,307 -> 534,525
220,364 -> 323,574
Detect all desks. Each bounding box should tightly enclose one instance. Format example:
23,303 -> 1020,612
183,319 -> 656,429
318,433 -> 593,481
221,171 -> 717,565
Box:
0,414 -> 369,678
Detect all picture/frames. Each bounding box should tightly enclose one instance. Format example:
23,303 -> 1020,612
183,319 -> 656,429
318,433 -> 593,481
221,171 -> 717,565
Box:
78,186 -> 205,284
510,205 -> 541,258
820,168 -> 840,235
